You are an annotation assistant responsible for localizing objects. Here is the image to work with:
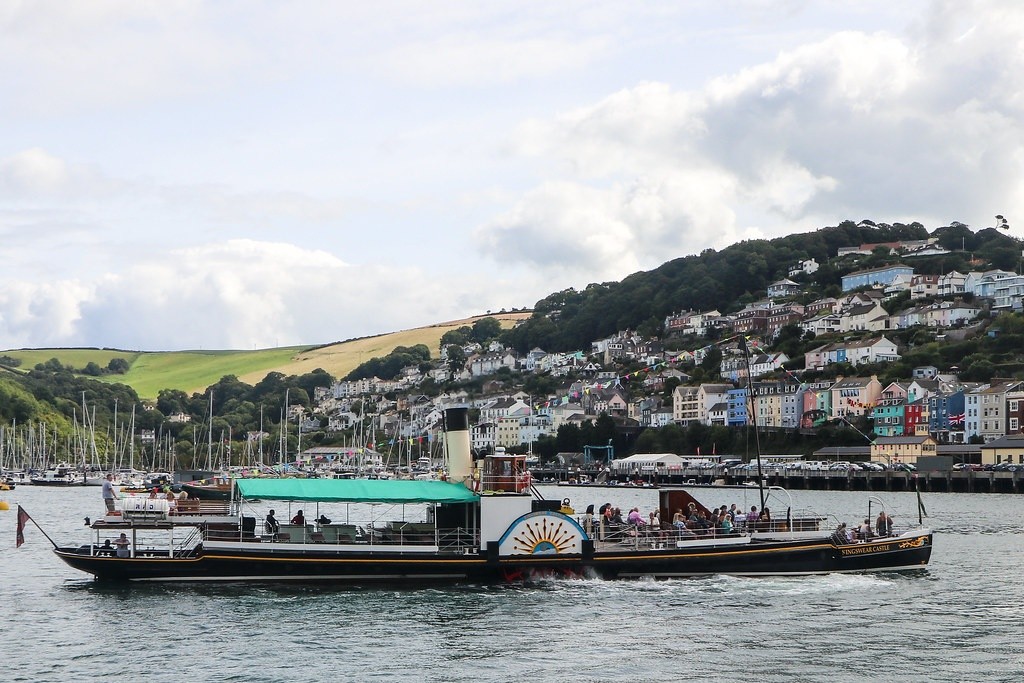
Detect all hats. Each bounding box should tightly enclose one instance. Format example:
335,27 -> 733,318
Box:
720,505 -> 727,510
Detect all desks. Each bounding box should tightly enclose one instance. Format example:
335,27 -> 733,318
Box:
280,524 -> 314,543
319,524 -> 357,543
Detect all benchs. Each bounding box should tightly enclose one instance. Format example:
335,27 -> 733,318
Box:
177,498 -> 232,516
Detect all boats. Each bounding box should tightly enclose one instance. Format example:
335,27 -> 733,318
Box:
478,445 -> 532,497
0,478 -> 16,490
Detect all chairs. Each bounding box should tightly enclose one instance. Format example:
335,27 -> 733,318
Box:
583,518 -> 771,540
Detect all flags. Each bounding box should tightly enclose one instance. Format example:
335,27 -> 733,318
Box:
947,411 -> 965,425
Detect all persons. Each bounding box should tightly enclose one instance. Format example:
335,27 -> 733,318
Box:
99,539 -> 115,556
113,533 -> 130,558
266,509 -> 279,543
102,474 -> 121,512
148,487 -> 190,516
583,502 -> 772,540
290,510 -> 307,524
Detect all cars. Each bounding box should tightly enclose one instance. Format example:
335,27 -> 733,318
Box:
714,457 -> 1024,473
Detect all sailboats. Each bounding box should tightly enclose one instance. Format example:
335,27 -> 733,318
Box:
0,387 -> 449,486
55,334 -> 933,587
